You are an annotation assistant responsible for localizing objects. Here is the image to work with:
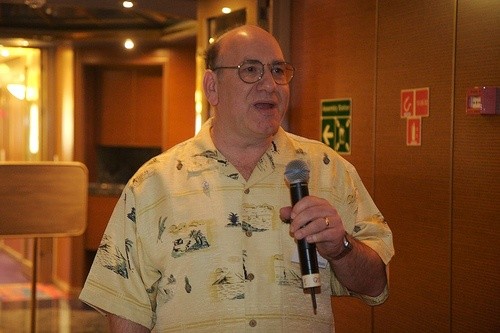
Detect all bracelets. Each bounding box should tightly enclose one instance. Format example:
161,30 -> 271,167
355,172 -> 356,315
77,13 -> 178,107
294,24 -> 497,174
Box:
336,230 -> 355,262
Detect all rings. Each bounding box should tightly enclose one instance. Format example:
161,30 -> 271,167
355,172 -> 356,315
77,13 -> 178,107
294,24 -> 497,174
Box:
324,216 -> 330,229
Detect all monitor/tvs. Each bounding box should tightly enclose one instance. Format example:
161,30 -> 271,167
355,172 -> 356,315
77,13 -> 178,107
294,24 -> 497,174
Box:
94,144 -> 162,186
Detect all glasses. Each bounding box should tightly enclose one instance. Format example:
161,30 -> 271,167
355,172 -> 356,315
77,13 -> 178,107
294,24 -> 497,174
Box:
214,61 -> 295,85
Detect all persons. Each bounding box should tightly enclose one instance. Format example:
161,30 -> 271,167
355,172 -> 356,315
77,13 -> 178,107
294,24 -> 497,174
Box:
78,23 -> 396,332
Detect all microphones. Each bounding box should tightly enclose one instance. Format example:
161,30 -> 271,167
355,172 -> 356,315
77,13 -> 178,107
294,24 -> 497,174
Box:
284,159 -> 321,309
